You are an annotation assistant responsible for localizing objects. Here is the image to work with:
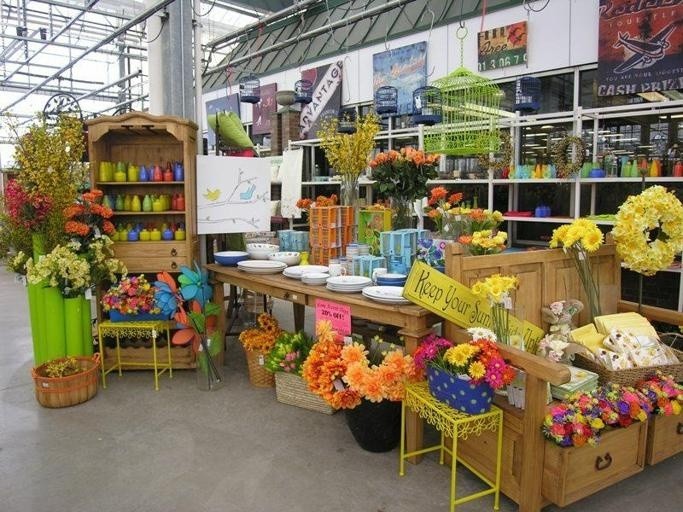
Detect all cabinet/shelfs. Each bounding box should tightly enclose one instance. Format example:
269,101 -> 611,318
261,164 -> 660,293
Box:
286,102 -> 683,333
87,111 -> 199,370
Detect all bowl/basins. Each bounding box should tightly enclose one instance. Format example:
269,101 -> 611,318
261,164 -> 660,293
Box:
269,251 -> 302,266
246,243 -> 280,260
213,251 -> 250,266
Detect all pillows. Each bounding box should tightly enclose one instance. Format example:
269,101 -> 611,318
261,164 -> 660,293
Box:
228,110 -> 244,133
206,111 -> 254,150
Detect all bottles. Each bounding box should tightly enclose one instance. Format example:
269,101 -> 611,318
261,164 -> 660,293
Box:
138,165 -> 150,181
131,195 -> 142,212
163,162 -> 173,181
114,161 -> 127,181
111,222 -> 185,241
115,194 -> 123,211
171,194 -> 184,211
127,163 -> 140,181
102,193 -> 115,211
124,193 -> 131,211
150,165 -> 163,181
173,162 -> 184,181
99,161 -> 116,181
142,193 -> 170,211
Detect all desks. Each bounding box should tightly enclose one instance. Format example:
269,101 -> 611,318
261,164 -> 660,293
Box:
203,264 -> 433,465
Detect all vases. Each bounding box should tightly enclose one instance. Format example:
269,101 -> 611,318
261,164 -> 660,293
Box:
345,395 -> 403,453
99,161 -> 183,182
672,160 -> 683,178
31,232 -> 45,364
535,206 -> 551,217
394,202 -> 413,230
340,179 -> 360,206
195,344 -> 224,392
112,222 -> 184,240
275,90 -> 297,112
43,285 -> 64,362
502,163 -> 574,181
428,364 -> 492,415
102,194 -> 184,211
620,157 -> 661,177
581,162 -> 605,178
63,296 -> 83,360
26,285 -> 39,364
82,300 -> 93,355
109,308 -> 168,322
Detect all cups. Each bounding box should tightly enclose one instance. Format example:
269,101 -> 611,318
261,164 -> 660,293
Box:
359,243 -> 374,256
329,265 -> 346,277
373,267 -> 387,283
346,243 -> 359,259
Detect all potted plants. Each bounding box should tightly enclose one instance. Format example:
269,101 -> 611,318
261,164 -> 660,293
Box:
31,351 -> 101,408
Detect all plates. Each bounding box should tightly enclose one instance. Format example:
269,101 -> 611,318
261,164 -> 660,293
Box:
376,273 -> 407,282
376,280 -> 407,287
237,267 -> 287,274
301,273 -> 328,286
326,276 -> 372,293
237,259 -> 287,270
283,265 -> 328,278
361,292 -> 414,305
362,285 -> 406,300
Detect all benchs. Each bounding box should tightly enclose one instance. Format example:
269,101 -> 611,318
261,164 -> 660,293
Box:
445,234 -> 683,512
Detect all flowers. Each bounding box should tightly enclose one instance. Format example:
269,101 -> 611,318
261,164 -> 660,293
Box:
541,382 -> 652,447
81,234 -> 129,288
474,132 -> 512,169
61,190 -> 115,236
3,178 -> 57,229
610,184 -> 683,276
367,146 -> 440,204
100,274 -> 160,316
633,369 -> 683,415
471,272 -> 521,342
154,259 -> 225,389
550,297 -> 585,340
3,111 -> 85,255
262,330 -> 316,377
314,102 -> 382,181
296,319 -> 414,409
549,136 -> 586,177
535,334 -> 568,361
239,312 -> 281,351
357,212 -> 387,256
414,325 -> 514,388
550,217 -> 605,316
295,194 -> 338,214
423,187 -> 505,239
414,238 -> 446,275
456,230 -> 508,254
7,252 -> 40,275
23,235 -> 91,297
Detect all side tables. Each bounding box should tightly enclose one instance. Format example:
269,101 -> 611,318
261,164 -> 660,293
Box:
400,381 -> 504,512
98,320 -> 174,390
